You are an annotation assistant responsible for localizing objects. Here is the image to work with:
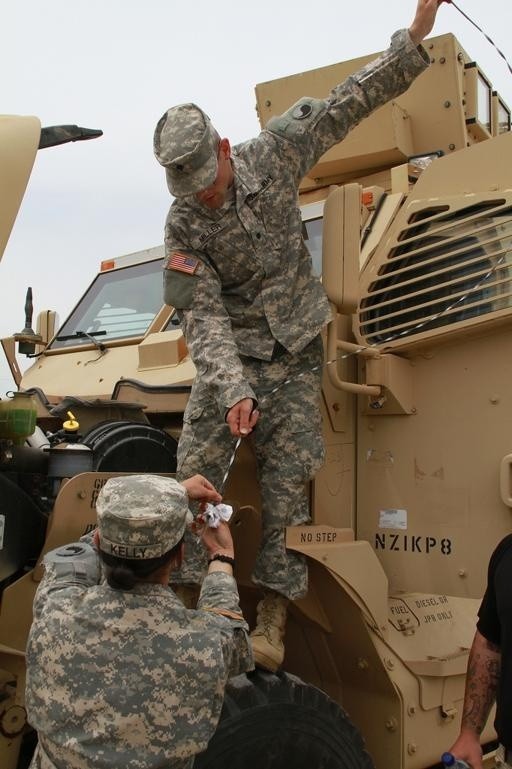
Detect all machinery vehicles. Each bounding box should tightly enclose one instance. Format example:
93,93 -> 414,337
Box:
1,0 -> 512,768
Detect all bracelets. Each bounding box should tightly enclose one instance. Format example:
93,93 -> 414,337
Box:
207,553 -> 235,568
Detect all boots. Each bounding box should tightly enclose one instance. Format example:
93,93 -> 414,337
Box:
248,598 -> 286,671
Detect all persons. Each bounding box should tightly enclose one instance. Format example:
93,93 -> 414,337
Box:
445,532 -> 512,769
23,475 -> 256,769
153,0 -> 444,672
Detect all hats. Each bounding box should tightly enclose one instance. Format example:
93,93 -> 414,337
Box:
96,475 -> 194,559
154,104 -> 218,197
